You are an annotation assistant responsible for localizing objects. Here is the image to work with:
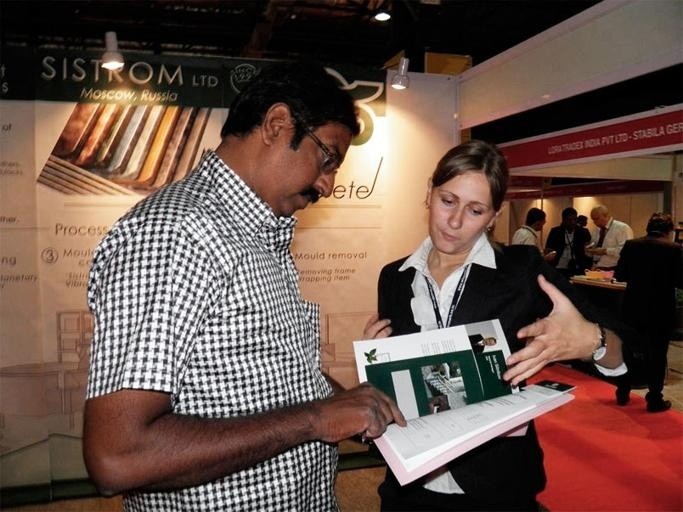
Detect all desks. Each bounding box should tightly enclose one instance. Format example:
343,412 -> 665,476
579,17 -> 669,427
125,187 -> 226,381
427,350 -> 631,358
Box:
570,275 -> 647,389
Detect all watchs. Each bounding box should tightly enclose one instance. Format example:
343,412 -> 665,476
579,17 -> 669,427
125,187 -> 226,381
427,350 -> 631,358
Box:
589,323 -> 607,363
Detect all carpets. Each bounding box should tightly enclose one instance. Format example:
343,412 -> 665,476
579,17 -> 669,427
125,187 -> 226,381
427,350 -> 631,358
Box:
524,364 -> 683,510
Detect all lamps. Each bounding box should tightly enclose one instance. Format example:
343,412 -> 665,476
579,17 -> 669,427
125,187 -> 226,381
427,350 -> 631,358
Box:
100,31 -> 125,70
390,58 -> 409,90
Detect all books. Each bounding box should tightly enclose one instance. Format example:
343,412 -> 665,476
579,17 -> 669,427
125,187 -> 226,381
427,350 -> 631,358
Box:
353,317 -> 576,487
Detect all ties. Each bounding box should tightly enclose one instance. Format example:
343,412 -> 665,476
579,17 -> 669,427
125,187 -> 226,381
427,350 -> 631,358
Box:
596,230 -> 604,247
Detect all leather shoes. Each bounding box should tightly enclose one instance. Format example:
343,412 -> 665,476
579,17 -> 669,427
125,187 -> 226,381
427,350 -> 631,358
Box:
645,400 -> 671,413
614,390 -> 630,406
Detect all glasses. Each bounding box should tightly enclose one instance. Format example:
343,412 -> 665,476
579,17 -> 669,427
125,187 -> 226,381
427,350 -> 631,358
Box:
293,110 -> 339,175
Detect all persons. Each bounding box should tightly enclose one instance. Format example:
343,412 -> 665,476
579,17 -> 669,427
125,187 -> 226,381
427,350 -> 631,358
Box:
468,334 -> 496,355
512,207 -> 556,261
576,216 -> 591,242
544,207 -> 593,279
363,139 -> 649,512
81,61 -> 406,512
584,205 -> 634,271
613,213 -> 683,412
